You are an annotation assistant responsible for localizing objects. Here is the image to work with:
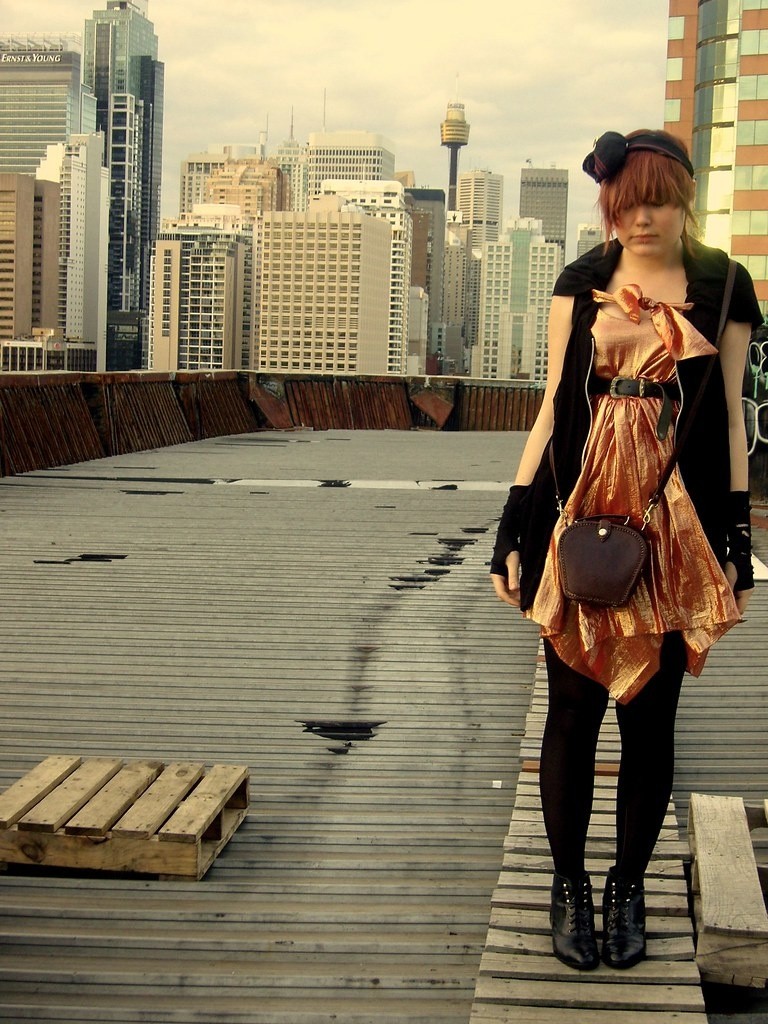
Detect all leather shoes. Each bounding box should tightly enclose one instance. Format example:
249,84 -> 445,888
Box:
603,872 -> 647,968
550,872 -> 599,969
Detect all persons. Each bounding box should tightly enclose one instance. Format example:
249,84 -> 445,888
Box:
488,128 -> 764,971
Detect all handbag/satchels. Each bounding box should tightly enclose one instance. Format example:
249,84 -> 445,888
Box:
559,514 -> 649,608
